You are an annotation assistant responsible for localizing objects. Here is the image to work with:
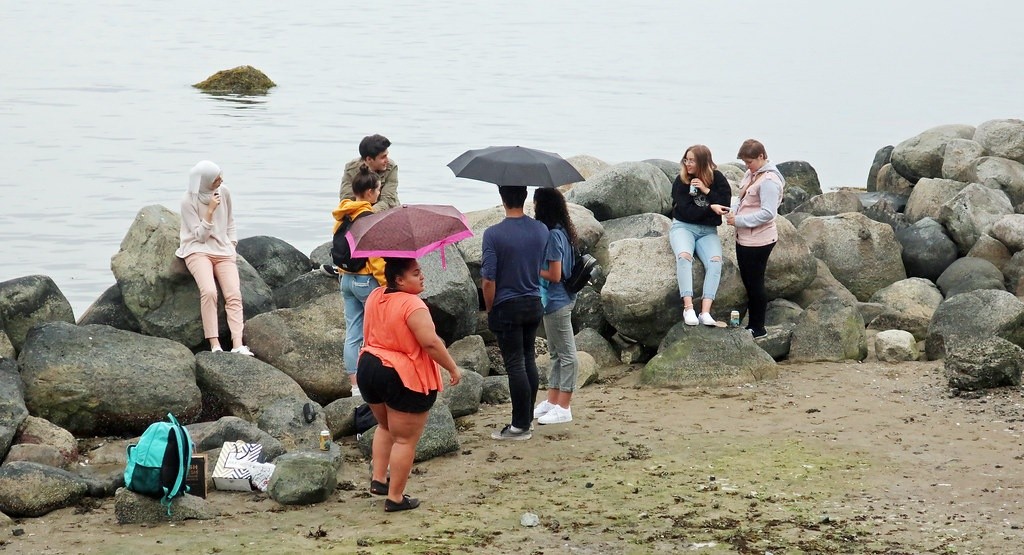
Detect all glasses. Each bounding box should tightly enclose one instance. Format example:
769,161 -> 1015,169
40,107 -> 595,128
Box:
682,158 -> 696,165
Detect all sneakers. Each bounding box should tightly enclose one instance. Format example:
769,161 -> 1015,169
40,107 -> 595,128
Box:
319,263 -> 344,277
533,400 -> 556,419
537,404 -> 573,424
697,312 -> 717,325
384,495 -> 419,512
682,307 -> 699,326
211,345 -> 224,354
230,345 -> 255,357
369,479 -> 389,495
490,422 -> 534,441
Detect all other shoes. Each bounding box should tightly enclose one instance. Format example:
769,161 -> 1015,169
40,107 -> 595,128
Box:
745,324 -> 768,339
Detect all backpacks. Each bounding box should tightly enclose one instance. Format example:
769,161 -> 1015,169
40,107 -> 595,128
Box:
125,409 -> 195,519
331,210 -> 376,273
550,225 -> 598,295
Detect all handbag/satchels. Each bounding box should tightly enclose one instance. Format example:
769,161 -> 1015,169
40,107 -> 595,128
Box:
212,438 -> 261,491
185,441 -> 208,500
354,402 -> 379,433
230,457 -> 275,493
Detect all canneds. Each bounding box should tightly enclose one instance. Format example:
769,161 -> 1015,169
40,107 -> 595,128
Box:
730,310 -> 740,328
689,179 -> 698,196
320,431 -> 331,450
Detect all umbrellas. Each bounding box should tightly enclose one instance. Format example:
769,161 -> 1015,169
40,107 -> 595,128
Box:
342,200 -> 477,270
447,143 -> 587,189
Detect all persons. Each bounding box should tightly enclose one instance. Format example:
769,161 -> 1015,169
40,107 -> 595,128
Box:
175,160 -> 257,360
331,162 -> 391,396
353,255 -> 463,513
668,141 -> 733,331
318,132 -> 401,276
727,139 -> 784,341
475,185 -> 553,442
532,187 -> 585,426
